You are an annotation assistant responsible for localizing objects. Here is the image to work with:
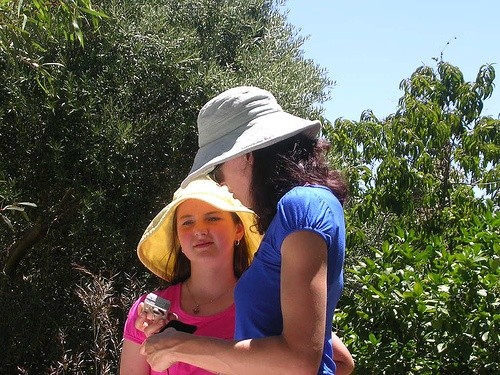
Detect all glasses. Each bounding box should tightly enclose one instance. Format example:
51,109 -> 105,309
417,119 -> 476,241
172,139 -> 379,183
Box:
206,161 -> 226,187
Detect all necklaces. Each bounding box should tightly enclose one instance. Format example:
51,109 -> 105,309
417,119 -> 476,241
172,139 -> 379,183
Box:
183,274 -> 237,314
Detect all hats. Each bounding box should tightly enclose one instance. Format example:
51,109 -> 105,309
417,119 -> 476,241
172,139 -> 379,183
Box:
137,178 -> 266,283
181,85 -> 324,190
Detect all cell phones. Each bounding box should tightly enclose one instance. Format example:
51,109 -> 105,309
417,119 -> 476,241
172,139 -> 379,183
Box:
159,320 -> 197,335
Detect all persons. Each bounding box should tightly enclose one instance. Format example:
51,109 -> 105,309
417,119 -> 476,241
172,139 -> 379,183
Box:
118,177 -> 356,375
135,86 -> 349,375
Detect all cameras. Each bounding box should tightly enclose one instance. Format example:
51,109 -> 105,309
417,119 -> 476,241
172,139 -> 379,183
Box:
143,293 -> 170,320
